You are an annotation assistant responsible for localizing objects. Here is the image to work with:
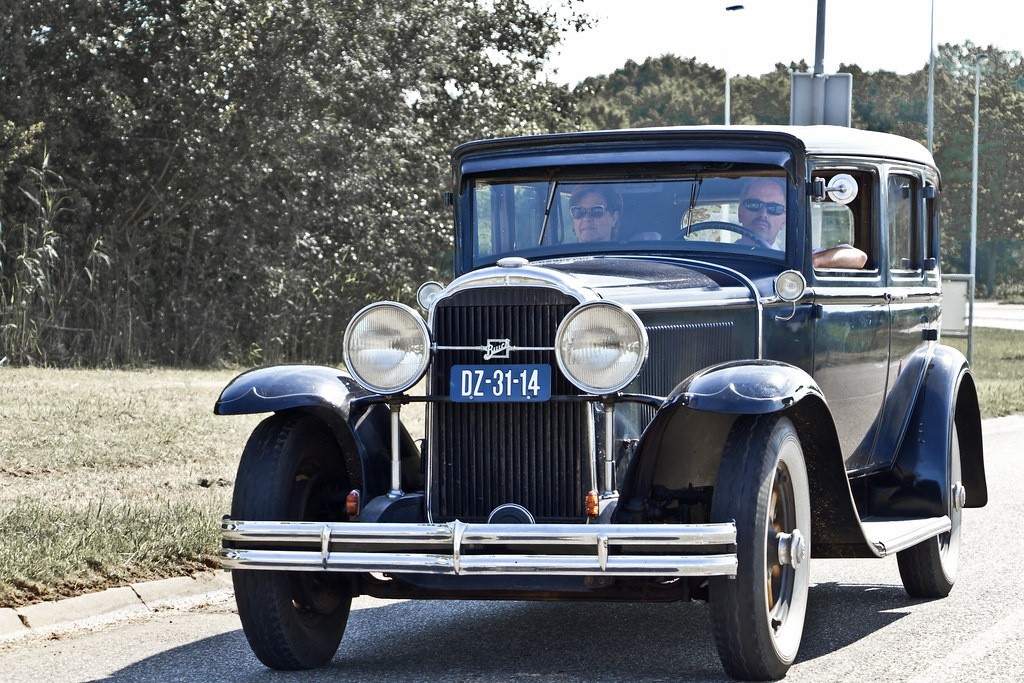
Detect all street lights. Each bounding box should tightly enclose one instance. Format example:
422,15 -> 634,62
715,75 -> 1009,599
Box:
967,54 -> 990,367
723,5 -> 746,126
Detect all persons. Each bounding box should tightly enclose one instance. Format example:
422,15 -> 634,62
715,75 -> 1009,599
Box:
569,186 -> 624,243
733,175 -> 868,269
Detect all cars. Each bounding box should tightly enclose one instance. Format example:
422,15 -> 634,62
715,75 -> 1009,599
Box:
214,124 -> 987,681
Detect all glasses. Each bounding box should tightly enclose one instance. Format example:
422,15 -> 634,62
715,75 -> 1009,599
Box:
740,199 -> 786,215
570,204 -> 609,219
